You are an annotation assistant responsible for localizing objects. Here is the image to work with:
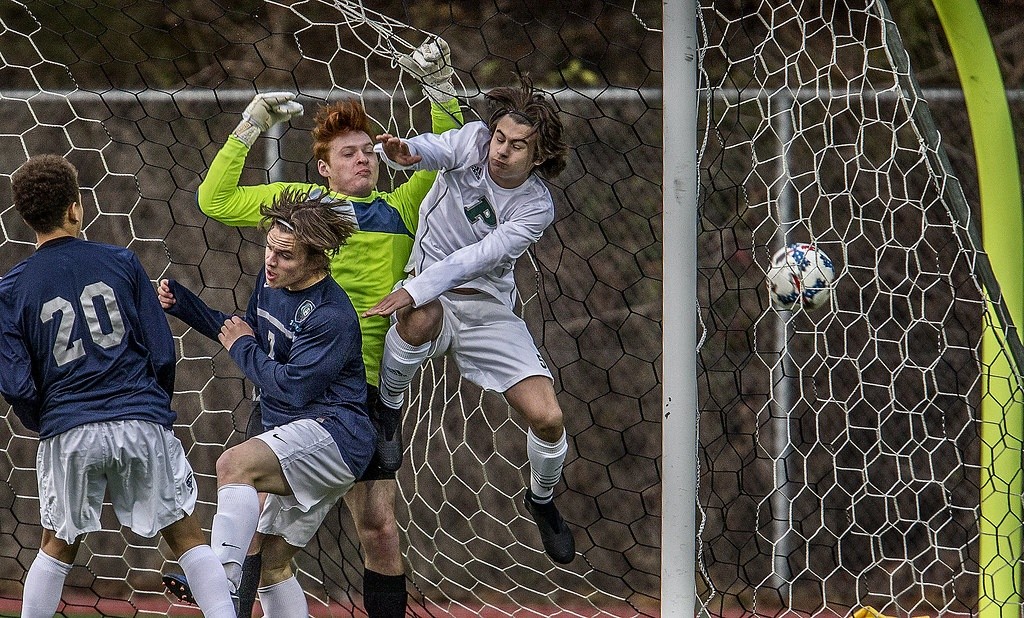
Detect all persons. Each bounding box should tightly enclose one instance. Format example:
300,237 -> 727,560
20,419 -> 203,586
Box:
156,192 -> 377,618
361,82 -> 576,564
0,154 -> 236,618
199,38 -> 464,618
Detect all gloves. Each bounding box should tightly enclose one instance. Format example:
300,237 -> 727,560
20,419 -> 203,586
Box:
241,91 -> 305,132
398,38 -> 451,84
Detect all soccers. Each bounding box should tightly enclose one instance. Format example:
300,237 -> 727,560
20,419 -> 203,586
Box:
765,242 -> 836,314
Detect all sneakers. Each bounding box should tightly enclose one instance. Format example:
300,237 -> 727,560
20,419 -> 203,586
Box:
373,398 -> 403,474
162,573 -> 241,615
523,487 -> 575,564
